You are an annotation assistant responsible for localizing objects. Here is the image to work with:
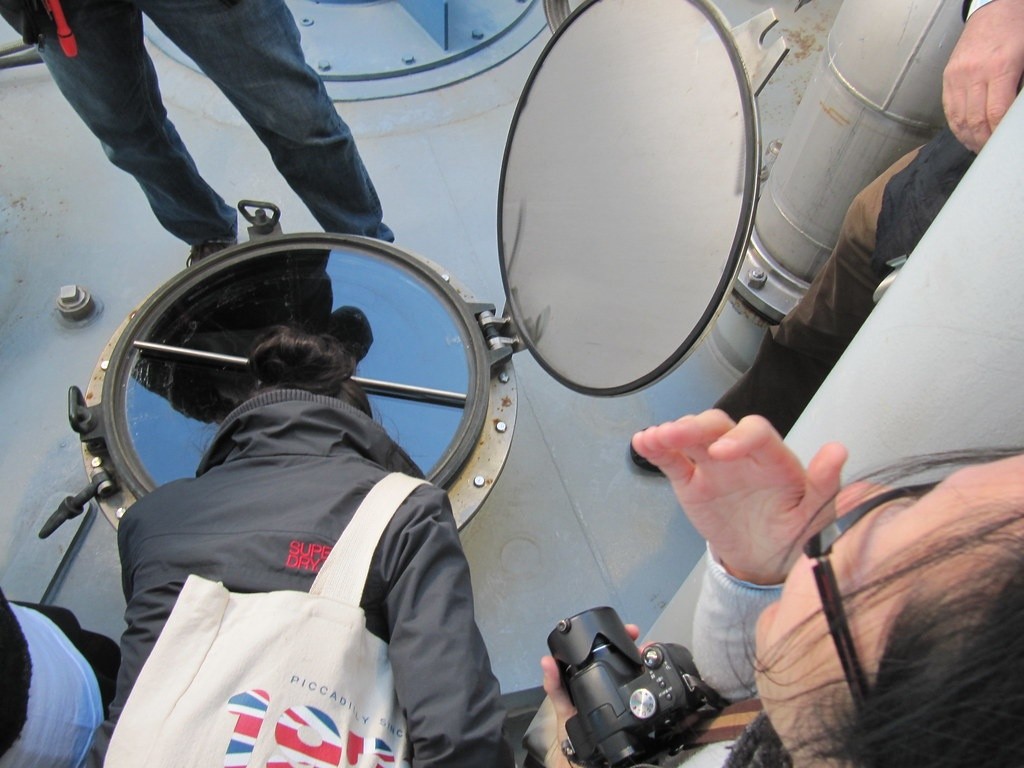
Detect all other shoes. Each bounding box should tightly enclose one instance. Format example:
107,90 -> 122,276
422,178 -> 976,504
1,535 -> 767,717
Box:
629,425 -> 674,473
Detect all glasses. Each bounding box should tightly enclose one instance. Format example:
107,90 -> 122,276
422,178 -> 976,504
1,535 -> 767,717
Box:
802,480 -> 944,713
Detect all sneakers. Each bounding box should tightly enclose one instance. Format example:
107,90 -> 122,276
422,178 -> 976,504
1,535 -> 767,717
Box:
185,236 -> 239,269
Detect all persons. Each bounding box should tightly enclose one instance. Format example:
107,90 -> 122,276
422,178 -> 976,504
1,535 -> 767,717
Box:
522,409 -> 1024,768
630,0 -> 1024,475
82,328 -> 515,768
0,0 -> 396,273
0,587 -> 121,768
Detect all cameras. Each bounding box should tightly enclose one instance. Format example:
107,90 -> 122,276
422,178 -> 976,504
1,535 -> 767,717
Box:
546,605 -> 706,768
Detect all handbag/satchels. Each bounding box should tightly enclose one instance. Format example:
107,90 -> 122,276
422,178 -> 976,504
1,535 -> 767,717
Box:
103,469 -> 435,768
